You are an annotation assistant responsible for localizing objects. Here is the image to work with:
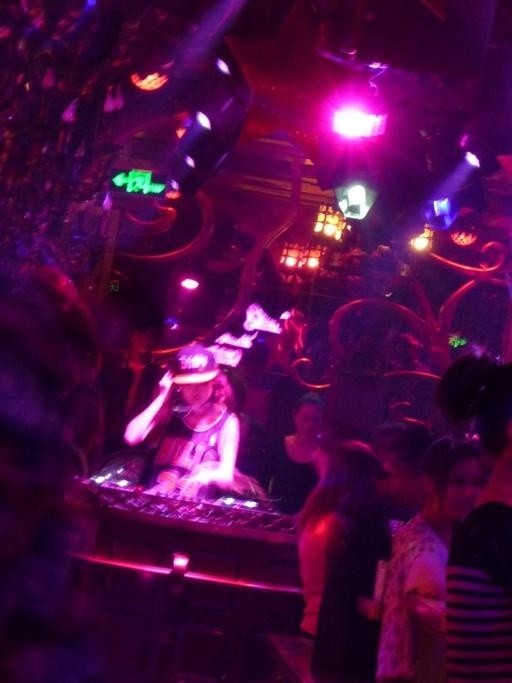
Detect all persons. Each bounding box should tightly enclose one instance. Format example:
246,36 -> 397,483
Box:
0,342 -> 511,682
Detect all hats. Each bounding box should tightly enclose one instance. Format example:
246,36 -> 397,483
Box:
172,346 -> 218,385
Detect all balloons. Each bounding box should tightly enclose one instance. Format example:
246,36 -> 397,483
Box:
0,1 -> 511,355
